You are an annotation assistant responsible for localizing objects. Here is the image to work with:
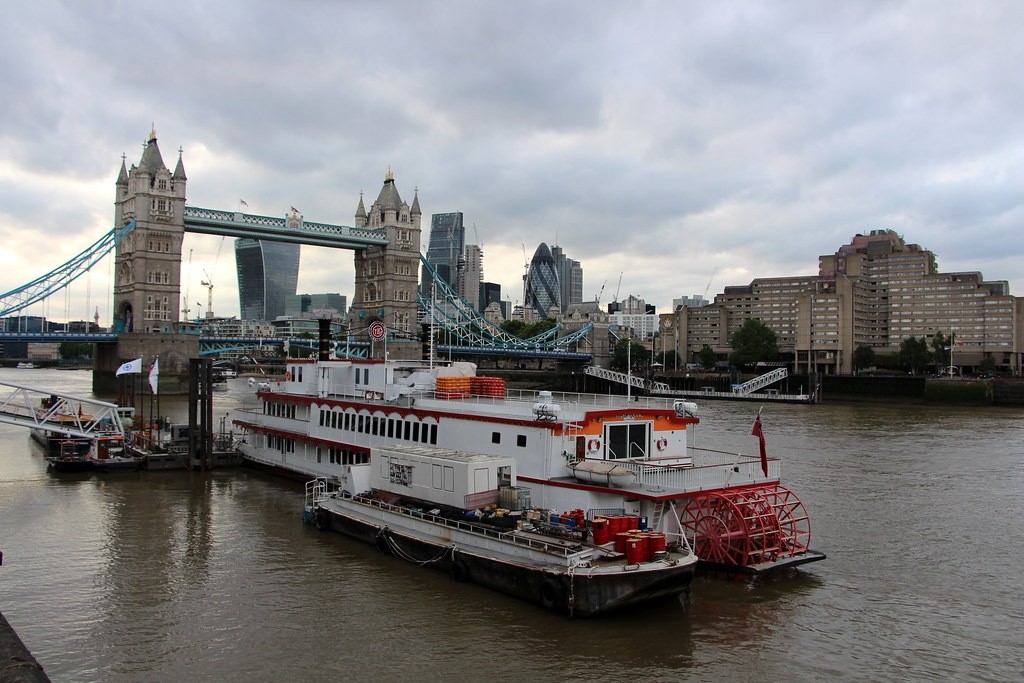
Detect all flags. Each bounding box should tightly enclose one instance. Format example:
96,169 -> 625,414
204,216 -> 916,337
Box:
148,357 -> 159,395
116,358 -> 141,377
751,411 -> 768,480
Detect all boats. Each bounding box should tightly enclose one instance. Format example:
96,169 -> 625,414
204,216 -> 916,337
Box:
231,336 -> 827,578
31,395 -> 68,447
16,361 -> 34,369
44,433 -> 150,474
303,442 -> 699,621
